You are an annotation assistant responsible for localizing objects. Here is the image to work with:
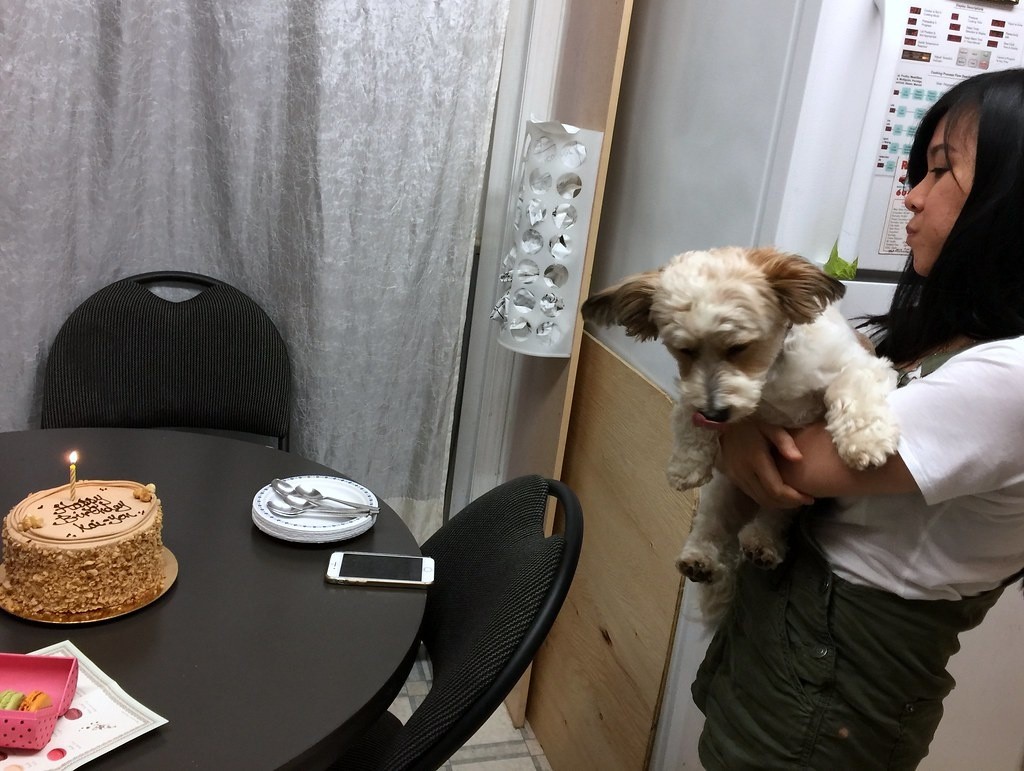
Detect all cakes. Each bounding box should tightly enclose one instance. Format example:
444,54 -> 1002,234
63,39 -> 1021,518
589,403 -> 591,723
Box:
1,479 -> 164,614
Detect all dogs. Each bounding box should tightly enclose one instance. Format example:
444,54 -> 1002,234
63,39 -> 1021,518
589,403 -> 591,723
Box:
579,244 -> 901,641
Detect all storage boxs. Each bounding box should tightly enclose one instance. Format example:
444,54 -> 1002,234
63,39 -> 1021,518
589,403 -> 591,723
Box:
0,653 -> 78,750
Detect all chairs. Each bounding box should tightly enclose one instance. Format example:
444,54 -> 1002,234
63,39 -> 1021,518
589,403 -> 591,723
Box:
42,271 -> 291,454
330,473 -> 585,771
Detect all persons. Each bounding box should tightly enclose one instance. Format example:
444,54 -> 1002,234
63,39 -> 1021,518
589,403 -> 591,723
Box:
690,69 -> 1023,771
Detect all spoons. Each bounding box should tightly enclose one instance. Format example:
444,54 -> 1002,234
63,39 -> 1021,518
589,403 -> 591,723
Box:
267,479 -> 381,518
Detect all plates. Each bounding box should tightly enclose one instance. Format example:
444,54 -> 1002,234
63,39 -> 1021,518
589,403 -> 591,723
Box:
0,546 -> 179,624
252,476 -> 380,543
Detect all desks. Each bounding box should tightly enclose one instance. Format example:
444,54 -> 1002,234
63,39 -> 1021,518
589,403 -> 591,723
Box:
0,427 -> 429,771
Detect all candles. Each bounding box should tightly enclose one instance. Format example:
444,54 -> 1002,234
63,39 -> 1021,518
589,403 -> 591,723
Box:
69,449 -> 79,501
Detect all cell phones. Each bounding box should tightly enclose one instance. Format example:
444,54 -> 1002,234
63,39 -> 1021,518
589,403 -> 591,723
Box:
325,551 -> 435,589
